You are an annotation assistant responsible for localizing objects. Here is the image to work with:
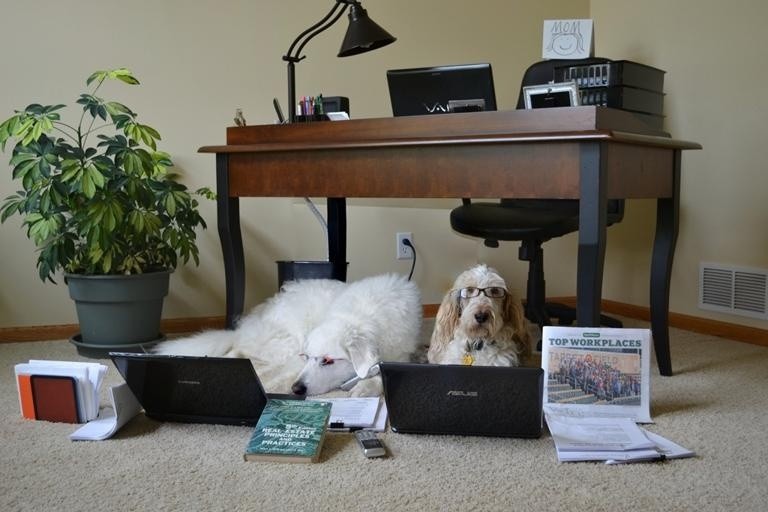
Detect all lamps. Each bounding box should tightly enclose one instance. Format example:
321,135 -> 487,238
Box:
282,0 -> 398,125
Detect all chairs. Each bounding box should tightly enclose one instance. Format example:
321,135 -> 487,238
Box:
449,57 -> 625,352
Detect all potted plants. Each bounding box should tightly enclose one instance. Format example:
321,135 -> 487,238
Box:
1,66 -> 217,359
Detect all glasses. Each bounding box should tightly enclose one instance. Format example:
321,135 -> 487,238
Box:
457,287 -> 509,300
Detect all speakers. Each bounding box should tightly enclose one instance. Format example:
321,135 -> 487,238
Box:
321,96 -> 350,119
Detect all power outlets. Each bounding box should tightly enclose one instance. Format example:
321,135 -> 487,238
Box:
397,231 -> 413,260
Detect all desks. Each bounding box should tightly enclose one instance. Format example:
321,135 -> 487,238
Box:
198,105 -> 703,376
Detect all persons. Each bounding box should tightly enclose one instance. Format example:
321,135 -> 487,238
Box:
563,358 -> 641,402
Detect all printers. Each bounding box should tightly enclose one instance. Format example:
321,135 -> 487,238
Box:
514,57 -> 667,117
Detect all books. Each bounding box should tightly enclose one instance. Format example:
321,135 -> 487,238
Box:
328,399 -> 391,433
243,397 -> 333,466
305,393 -> 380,429
542,407 -> 697,466
542,86 -> 671,119
554,60 -> 669,96
14,355 -> 144,441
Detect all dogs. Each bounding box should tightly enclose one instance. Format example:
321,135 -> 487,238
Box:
150,272 -> 424,398
427,263 -> 541,367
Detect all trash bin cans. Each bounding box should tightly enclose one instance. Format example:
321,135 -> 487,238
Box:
275,260 -> 350,292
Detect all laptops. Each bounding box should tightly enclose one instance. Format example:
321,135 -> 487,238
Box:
378,360 -> 545,440
107,351 -> 307,427
385,63 -> 498,117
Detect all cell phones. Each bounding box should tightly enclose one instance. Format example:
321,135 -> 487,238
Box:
352,430 -> 386,458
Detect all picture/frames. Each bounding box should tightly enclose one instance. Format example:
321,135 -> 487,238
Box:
522,80 -> 581,109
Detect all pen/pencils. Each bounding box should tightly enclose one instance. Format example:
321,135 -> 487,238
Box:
297,93 -> 324,115
